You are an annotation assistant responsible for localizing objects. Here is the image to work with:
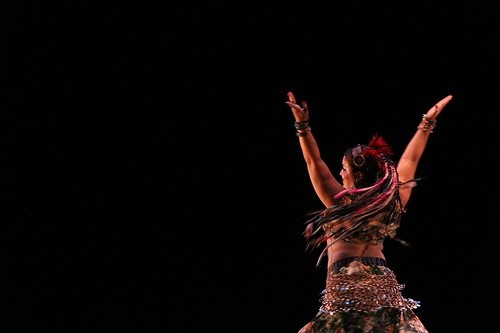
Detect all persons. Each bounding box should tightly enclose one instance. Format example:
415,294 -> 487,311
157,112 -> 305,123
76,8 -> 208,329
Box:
285,92 -> 453,333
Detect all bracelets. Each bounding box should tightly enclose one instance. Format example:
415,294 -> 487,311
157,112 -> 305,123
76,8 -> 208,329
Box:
418,114 -> 437,134
295,119 -> 311,136
